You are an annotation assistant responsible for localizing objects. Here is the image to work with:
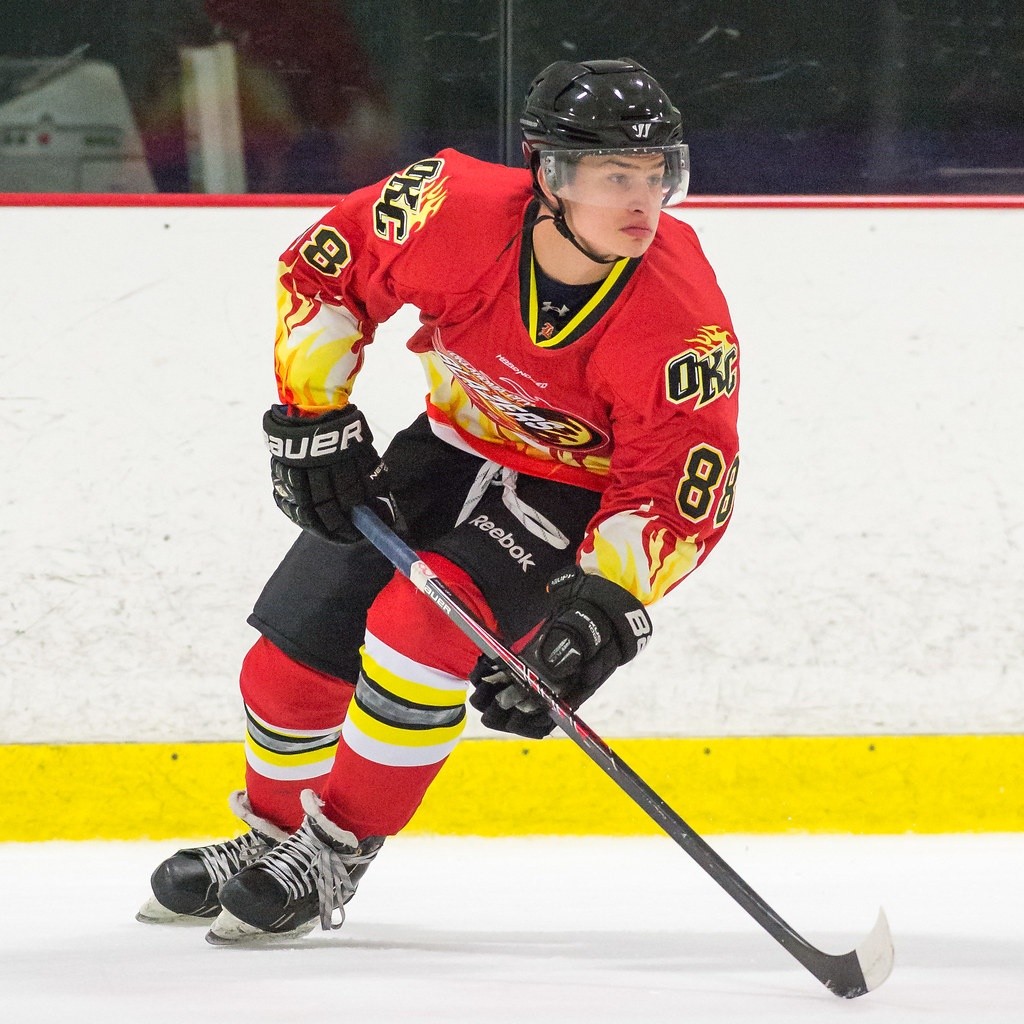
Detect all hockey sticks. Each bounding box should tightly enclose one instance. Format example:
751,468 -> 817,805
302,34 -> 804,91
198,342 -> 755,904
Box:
355,505 -> 895,1000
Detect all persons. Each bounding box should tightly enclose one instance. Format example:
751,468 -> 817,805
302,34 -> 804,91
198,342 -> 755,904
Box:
135,57 -> 739,948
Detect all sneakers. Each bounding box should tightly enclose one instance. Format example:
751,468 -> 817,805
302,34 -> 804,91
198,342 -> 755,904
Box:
205,789 -> 387,945
135,790 -> 290,924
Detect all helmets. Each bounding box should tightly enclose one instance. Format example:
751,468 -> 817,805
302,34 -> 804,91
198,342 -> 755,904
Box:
521,58 -> 690,207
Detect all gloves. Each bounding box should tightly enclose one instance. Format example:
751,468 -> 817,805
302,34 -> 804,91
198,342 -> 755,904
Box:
262,404 -> 398,543
468,565 -> 653,740
